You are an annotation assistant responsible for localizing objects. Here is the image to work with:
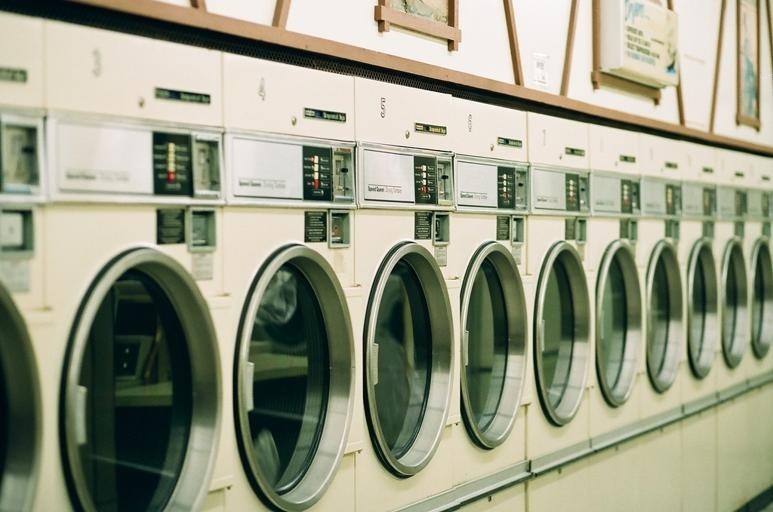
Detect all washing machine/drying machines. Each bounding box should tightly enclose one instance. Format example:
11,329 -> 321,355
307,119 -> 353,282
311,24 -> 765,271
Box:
0,8 -> 773,511
